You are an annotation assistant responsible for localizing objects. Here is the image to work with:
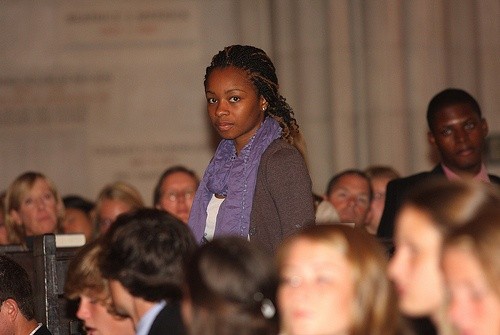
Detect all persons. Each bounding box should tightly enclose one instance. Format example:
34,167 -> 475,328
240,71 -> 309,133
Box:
375,89 -> 500,257
0,164 -> 499,335
187,45 -> 316,252
